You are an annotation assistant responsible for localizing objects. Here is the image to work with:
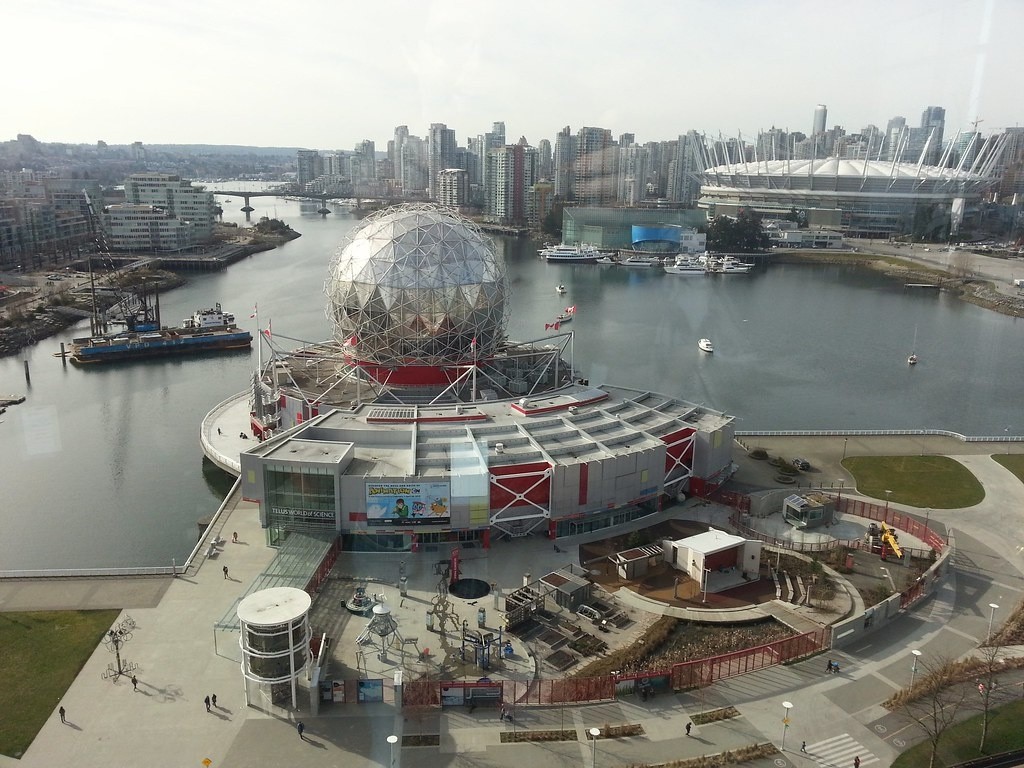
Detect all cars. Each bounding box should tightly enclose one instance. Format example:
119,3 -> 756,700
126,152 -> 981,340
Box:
48,275 -> 62,280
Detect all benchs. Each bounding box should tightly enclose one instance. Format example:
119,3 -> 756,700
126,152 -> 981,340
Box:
347,599 -> 375,615
204,535 -> 225,558
770,567 -> 806,603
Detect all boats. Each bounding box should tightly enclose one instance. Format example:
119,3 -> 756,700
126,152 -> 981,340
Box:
556,306 -> 574,323
664,258 -> 708,275
68,302 -> 253,364
663,251 -> 755,273
596,257 -> 616,264
617,255 -> 660,266
537,240 -> 615,263
908,353 -> 917,364
556,280 -> 567,293
698,339 -> 713,352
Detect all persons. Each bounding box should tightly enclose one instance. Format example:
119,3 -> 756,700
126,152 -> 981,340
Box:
825,659 -> 841,673
800,741 -> 806,753
297,720 -> 305,740
500,703 -> 514,723
204,694 -> 217,712
223,565 -> 228,579
854,756 -> 860,768
59,705 -> 66,723
232,532 -> 238,544
217,427 -> 221,436
685,722 -> 692,737
132,675 -> 137,691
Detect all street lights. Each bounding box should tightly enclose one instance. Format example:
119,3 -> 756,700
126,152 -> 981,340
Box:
1004,427 -> 1012,455
883,490 -> 892,523
835,479 -> 846,511
908,650 -> 922,695
18,266 -> 22,286
920,426 -> 927,456
589,728 -> 600,768
843,438 -> 848,459
922,508 -> 933,542
66,268 -> 69,280
985,604 -> 999,647
386,735 -> 398,768
779,702 -> 793,751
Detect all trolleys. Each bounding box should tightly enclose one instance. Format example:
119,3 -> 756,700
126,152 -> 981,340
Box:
503,714 -> 513,722
831,663 -> 840,673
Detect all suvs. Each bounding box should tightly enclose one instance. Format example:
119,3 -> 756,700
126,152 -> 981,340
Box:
792,458 -> 810,470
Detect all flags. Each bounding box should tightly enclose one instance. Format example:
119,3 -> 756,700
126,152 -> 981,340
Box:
251,303 -> 271,339
343,335 -> 357,347
472,337 -> 476,345
544,321 -> 560,330
566,306 -> 577,313
